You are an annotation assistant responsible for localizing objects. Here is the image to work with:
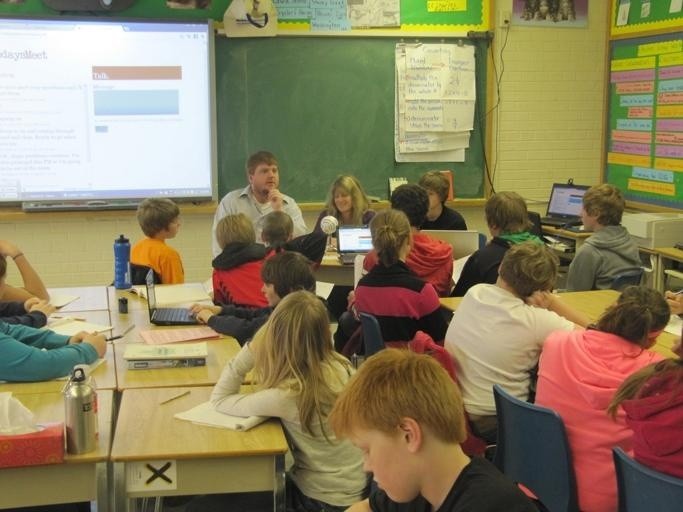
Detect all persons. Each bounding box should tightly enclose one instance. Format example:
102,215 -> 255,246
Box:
517,286 -> 671,512
129,196 -> 185,284
563,183 -> 642,292
0,237 -> 108,384
444,243 -> 591,447
332,349 -> 548,512
352,184 -> 457,342
212,153 -> 307,259
209,290 -> 368,512
416,171 -> 466,231
607,324 -> 683,480
450,191 -> 550,296
193,211 -> 316,349
313,176 -> 377,258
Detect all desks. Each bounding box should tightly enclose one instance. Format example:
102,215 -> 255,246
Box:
0,226 -> 683,512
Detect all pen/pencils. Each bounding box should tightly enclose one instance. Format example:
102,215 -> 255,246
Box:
665,290 -> 683,299
123,324 -> 135,335
161,391 -> 191,405
106,336 -> 123,342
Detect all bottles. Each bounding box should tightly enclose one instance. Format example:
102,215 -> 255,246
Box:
112,234 -> 131,290
62,366 -> 100,455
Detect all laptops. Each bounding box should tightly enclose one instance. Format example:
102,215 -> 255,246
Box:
145,268 -> 205,324
336,224 -> 374,266
539,183 -> 591,228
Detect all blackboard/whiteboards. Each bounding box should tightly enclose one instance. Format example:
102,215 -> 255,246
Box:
0,28 -> 495,221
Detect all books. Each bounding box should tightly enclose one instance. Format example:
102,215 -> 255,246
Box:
393,42 -> 477,163
123,326 -> 225,369
174,398 -> 271,433
129,281 -> 216,308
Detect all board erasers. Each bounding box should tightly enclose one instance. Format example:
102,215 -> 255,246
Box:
84,200 -> 109,206
